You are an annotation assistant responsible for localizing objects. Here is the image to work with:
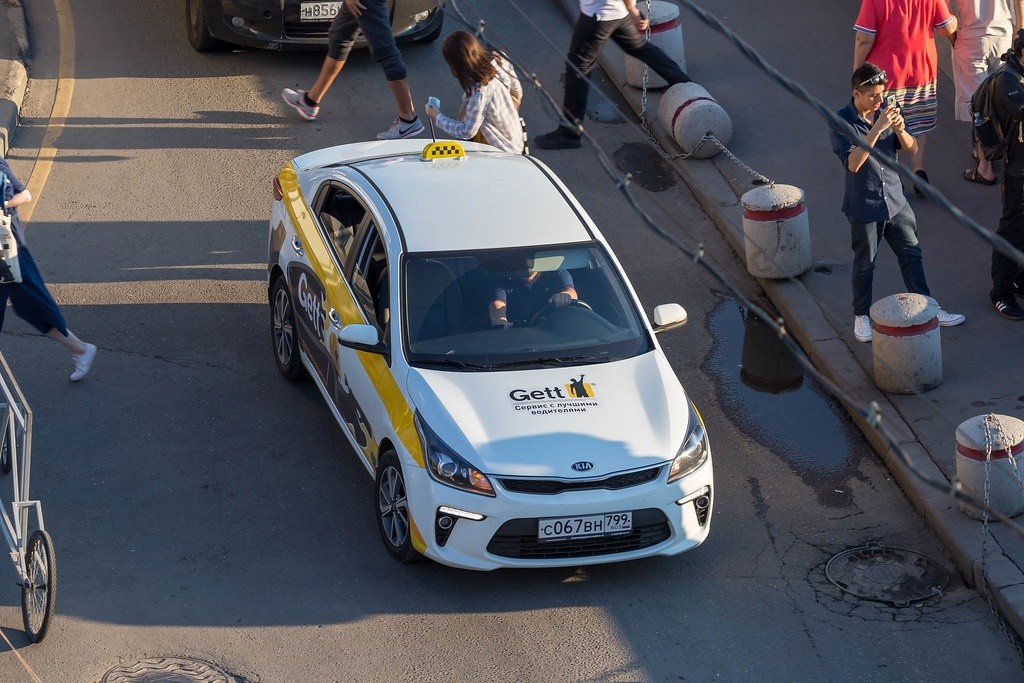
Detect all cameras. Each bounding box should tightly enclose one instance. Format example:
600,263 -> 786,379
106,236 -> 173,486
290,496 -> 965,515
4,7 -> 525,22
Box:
429,97 -> 441,111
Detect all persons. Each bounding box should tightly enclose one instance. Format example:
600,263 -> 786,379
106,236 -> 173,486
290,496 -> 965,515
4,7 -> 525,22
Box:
489,249 -> 578,330
375,258 -> 446,340
534,0 -> 694,151
943,0 -> 1024,184
829,62 -> 966,342
0,156 -> 98,381
989,28 -> 1024,321
425,30 -> 524,156
282,0 -> 426,141
853,0 -> 957,197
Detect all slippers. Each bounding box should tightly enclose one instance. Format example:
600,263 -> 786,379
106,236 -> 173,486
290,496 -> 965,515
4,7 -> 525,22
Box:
963,165 -> 997,185
971,149 -> 981,162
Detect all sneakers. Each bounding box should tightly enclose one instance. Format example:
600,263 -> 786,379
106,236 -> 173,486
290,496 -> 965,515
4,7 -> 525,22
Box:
376,117 -> 426,140
281,88 -> 320,121
936,307 -> 965,327
853,315 -> 872,342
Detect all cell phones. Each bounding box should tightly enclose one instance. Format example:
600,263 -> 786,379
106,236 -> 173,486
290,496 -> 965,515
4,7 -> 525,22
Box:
886,94 -> 896,110
639,10 -> 647,21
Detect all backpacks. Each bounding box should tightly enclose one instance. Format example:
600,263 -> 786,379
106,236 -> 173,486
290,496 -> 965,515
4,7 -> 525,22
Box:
968,53 -> 1024,161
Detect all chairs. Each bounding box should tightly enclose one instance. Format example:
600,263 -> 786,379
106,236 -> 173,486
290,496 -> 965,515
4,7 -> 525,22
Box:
377,259 -> 469,343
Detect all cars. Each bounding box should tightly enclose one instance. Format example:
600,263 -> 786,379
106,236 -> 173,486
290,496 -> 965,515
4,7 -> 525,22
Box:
187,0 -> 447,54
265,139 -> 716,573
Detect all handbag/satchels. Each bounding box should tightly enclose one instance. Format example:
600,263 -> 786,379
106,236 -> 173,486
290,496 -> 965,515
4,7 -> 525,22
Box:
0,207 -> 22,282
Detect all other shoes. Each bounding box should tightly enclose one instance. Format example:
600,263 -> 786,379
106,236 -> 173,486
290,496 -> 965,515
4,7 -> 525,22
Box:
1013,273 -> 1024,295
70,343 -> 97,382
990,293 -> 1024,320
532,130 -> 581,150
914,170 -> 929,194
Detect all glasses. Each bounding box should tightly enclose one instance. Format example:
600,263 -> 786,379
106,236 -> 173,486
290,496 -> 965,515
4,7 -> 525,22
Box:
854,70 -> 887,89
